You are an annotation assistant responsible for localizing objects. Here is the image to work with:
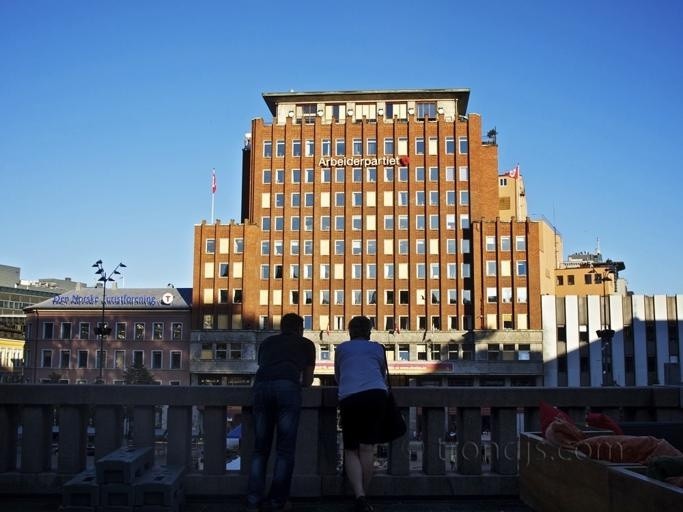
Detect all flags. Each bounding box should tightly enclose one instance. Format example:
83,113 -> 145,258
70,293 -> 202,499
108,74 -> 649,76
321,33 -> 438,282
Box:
210,168 -> 217,195
505,165 -> 518,179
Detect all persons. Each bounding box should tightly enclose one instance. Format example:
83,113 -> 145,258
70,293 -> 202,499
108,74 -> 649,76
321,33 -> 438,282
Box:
241,311 -> 316,512
332,314 -> 395,512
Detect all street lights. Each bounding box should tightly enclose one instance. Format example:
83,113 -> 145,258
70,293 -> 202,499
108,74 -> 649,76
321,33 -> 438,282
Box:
90,260 -> 126,385
23,309 -> 38,383
587,263 -> 618,386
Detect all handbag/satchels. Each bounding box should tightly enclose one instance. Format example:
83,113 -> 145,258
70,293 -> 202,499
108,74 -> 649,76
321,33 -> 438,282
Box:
371,389 -> 407,445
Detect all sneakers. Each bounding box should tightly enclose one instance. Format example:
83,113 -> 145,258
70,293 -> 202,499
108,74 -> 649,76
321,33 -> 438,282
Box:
239,496 -> 263,512
264,498 -> 296,512
355,494 -> 380,512
